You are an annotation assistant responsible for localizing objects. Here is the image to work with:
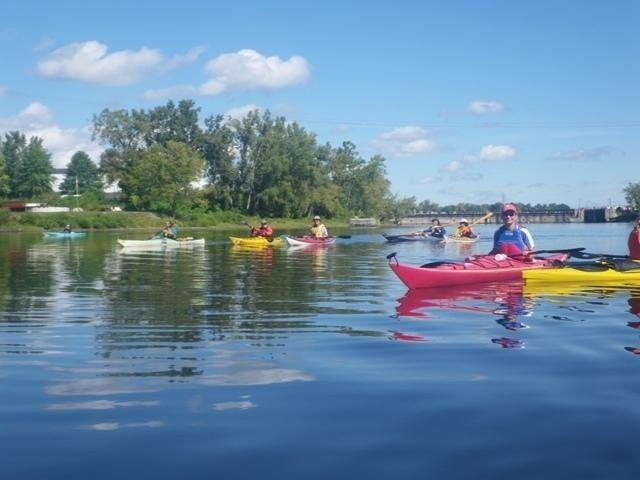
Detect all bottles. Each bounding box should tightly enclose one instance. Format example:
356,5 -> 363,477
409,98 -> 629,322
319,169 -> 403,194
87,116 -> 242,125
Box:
495,253 -> 507,262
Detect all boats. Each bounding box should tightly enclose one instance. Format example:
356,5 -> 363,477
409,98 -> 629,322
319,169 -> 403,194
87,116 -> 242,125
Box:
521,258 -> 640,286
117,238 -> 205,249
381,232 -> 443,241
43,232 -> 87,241
386,252 -> 570,290
229,235 -> 289,247
444,232 -> 480,242
286,235 -> 336,245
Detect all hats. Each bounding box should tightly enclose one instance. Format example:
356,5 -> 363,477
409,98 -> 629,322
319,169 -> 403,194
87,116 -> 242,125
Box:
313,216 -> 320,220
503,204 -> 516,213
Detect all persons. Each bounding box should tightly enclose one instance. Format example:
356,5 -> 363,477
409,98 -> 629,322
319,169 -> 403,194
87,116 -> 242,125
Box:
457,218 -> 477,238
491,292 -> 536,353
159,220 -> 178,240
627,217 -> 640,261
486,201 -> 538,258
625,291 -> 640,356
423,218 -> 446,237
301,215 -> 329,238
252,218 -> 273,237
